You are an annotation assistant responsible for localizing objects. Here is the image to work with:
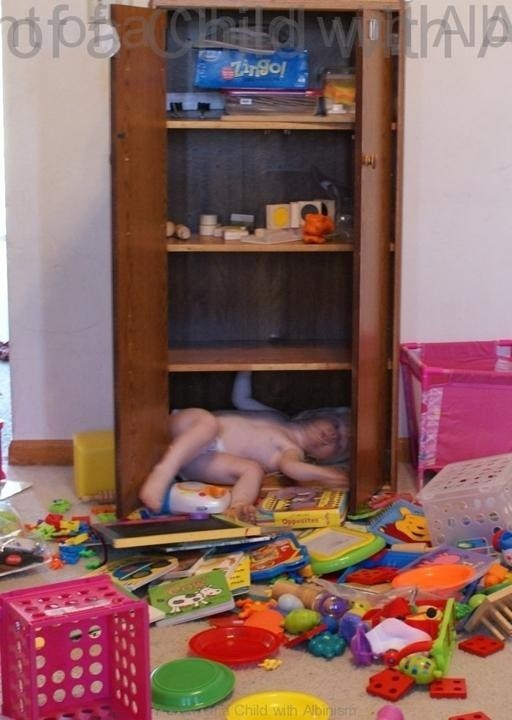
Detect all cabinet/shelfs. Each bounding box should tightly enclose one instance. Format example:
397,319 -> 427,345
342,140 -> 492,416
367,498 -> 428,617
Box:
109,1 -> 409,513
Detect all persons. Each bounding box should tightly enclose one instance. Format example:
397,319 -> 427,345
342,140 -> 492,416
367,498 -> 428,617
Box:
0,481 -> 512,720
139,371 -> 351,523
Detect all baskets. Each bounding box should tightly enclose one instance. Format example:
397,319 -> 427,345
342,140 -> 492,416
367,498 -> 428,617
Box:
0,572 -> 152,720
415,450 -> 512,556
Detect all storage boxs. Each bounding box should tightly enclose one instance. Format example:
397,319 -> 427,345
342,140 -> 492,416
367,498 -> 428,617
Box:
397,340 -> 512,497
189,41 -> 311,89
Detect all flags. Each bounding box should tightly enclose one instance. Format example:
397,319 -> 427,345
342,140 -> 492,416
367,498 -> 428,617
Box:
0,481 -> 512,720
